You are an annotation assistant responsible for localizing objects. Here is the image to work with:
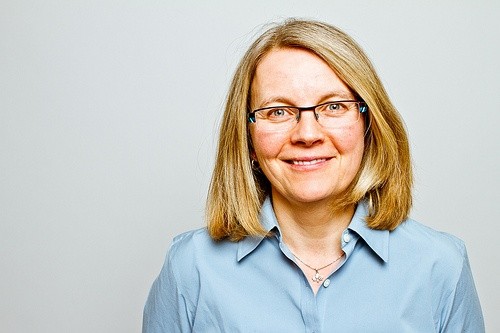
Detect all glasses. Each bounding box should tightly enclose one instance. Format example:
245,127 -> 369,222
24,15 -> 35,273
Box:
248,97 -> 363,128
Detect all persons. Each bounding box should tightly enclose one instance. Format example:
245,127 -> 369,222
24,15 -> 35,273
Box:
142,18 -> 485,333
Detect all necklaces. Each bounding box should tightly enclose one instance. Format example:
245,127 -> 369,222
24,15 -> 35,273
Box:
290,249 -> 346,284
